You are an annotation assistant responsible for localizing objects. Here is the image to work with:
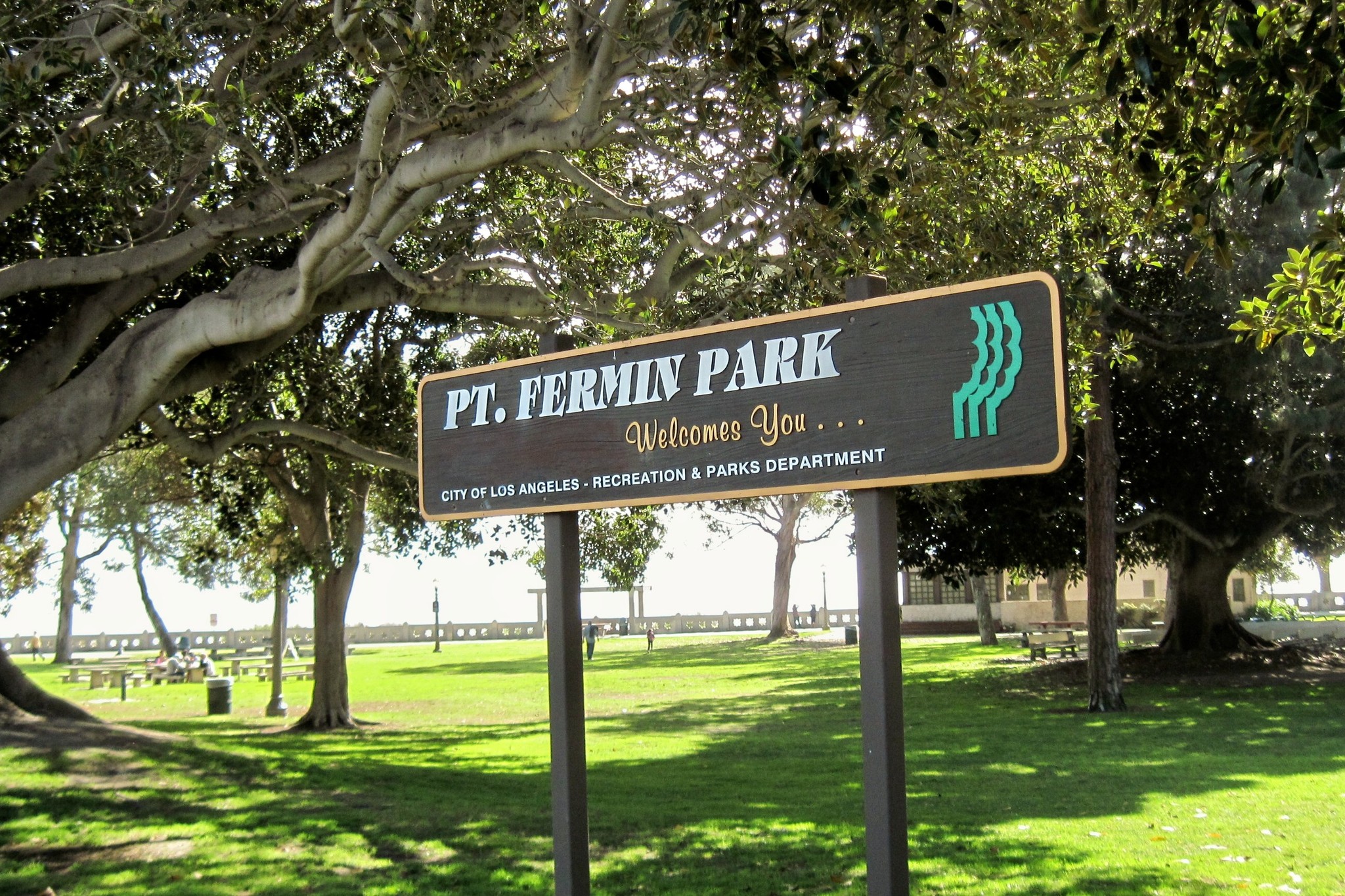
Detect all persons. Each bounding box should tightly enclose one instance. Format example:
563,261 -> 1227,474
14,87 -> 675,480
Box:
792,604 -> 802,628
647,628 -> 656,654
155,650 -> 219,677
583,621 -> 599,661
810,604 -> 817,628
28,631 -> 45,662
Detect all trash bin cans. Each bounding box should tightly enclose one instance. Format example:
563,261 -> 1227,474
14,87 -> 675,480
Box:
845,626 -> 859,643
206,678 -> 233,714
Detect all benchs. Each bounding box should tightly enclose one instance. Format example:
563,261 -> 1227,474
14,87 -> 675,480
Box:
1030,641 -> 1076,661
1019,630 -> 1075,647
71,658 -> 85,664
1151,622 -> 1164,630
150,674 -> 183,685
99,657 -> 130,664
1028,622 -> 1084,629
219,665 -> 317,681
59,673 -> 111,684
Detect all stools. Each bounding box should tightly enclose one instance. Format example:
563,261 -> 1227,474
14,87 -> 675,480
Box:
129,674 -> 145,687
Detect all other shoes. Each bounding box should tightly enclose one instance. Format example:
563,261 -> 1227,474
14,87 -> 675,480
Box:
648,651 -> 650,653
588,658 -> 593,660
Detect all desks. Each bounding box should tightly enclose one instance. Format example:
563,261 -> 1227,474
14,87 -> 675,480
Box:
62,665 -> 127,689
223,654 -> 273,675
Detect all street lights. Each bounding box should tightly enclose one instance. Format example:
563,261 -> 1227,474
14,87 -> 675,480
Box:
821,564 -> 831,631
433,578 -> 442,652
